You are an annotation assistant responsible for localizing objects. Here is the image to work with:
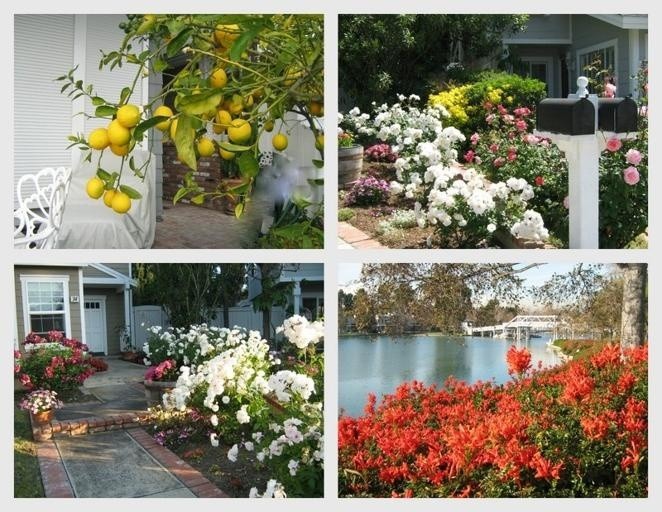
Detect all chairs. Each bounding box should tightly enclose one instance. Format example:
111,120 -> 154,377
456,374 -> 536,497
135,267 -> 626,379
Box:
14,166 -> 72,249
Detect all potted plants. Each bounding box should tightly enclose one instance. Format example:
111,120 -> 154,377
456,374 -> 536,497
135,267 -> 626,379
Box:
338,130 -> 365,190
143,359 -> 181,407
18,390 -> 64,425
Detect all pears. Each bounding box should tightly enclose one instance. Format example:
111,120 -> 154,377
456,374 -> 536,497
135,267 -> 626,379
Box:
85,22 -> 323,212
